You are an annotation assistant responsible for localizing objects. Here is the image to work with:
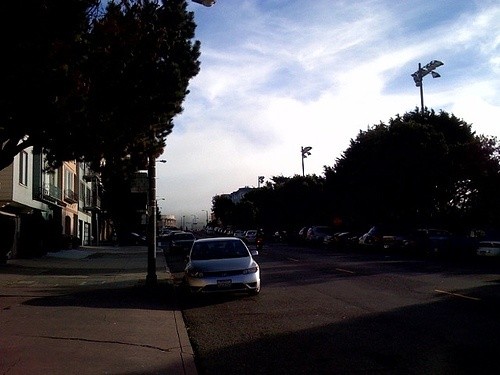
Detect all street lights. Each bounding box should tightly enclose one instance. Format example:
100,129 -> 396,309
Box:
301,146 -> 312,176
411,60 -> 444,114
258,176 -> 264,188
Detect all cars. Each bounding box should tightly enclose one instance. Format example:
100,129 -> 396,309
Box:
184,237 -> 261,296
157,229 -> 173,244
171,232 -> 194,251
299,225 -> 409,248
476,241 -> 500,256
206,227 -> 258,245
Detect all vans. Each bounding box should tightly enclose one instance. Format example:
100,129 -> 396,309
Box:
419,229 -> 466,254
